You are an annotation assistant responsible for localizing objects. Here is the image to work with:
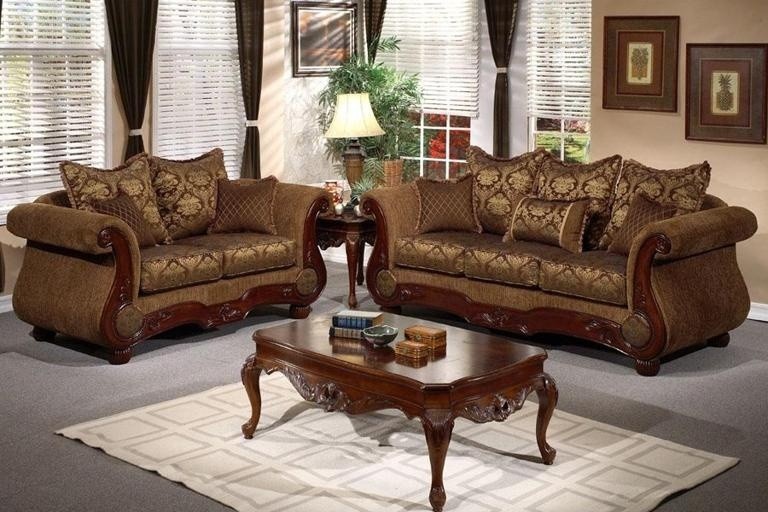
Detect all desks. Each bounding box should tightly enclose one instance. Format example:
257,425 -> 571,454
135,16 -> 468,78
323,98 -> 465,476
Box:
240,309 -> 560,510
316,204 -> 376,308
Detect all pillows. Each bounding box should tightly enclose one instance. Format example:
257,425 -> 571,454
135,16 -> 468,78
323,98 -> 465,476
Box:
609,194 -> 675,254
146,148 -> 229,240
58,151 -> 173,252
594,158 -> 712,256
531,150 -> 622,255
210,175 -> 280,237
87,185 -> 155,249
465,145 -> 544,242
502,191 -> 591,255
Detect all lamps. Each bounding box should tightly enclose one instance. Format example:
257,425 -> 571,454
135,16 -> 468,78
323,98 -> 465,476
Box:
323,92 -> 384,198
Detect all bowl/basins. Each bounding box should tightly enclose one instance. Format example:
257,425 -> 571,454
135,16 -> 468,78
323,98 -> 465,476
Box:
362,324 -> 399,350
364,346 -> 396,364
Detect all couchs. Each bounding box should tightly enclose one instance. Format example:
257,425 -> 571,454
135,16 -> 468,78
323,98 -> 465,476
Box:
359,146 -> 758,371
6,147 -> 334,366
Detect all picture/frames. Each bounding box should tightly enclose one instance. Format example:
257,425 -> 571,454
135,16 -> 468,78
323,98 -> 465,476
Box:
291,1 -> 357,77
602,15 -> 680,113
686,43 -> 767,144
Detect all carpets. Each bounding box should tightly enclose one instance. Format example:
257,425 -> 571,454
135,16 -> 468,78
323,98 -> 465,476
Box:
54,371 -> 741,510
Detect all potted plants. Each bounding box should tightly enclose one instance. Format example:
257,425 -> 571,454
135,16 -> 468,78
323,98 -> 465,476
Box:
318,36 -> 419,186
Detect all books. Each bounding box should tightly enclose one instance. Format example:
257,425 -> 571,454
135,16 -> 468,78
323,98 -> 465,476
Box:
332,310 -> 383,330
330,337 -> 374,354
329,325 -> 381,340
